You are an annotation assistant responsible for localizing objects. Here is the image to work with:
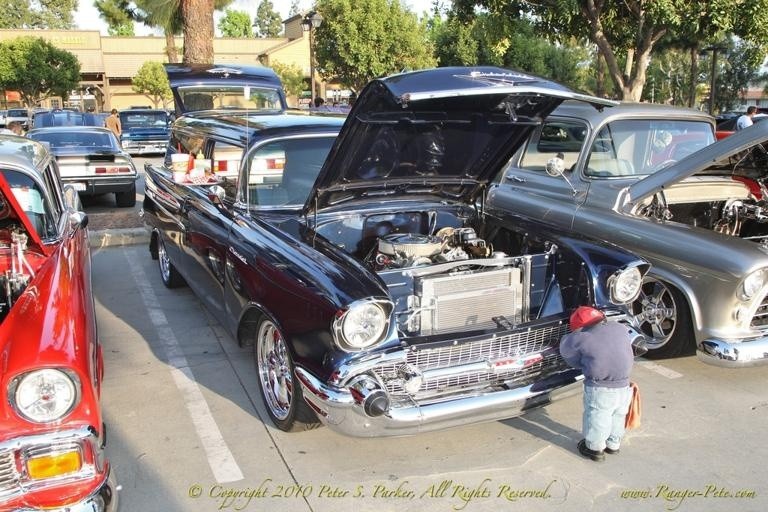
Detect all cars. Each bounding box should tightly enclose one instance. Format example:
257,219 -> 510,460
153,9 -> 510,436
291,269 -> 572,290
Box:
140,63 -> 768,438
0,108 -> 140,511
118,108 -> 173,157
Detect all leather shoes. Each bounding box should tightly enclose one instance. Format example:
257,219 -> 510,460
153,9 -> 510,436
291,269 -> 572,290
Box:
579,442 -> 606,462
604,447 -> 620,455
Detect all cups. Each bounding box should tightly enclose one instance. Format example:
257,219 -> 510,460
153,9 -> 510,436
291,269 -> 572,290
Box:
170,154 -> 189,183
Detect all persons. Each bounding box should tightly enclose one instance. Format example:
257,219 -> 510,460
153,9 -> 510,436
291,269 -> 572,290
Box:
8,122 -> 22,136
105,109 -> 121,142
560,306 -> 634,462
309,98 -> 356,109
737,107 -> 757,131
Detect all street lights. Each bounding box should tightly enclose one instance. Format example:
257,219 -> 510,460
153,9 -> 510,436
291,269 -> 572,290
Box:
299,11 -> 324,106
696,46 -> 730,116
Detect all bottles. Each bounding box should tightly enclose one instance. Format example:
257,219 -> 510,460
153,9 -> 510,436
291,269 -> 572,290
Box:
188,149 -> 204,174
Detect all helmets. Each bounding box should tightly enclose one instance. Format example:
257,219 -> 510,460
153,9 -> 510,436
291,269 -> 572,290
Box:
568,305 -> 605,331
